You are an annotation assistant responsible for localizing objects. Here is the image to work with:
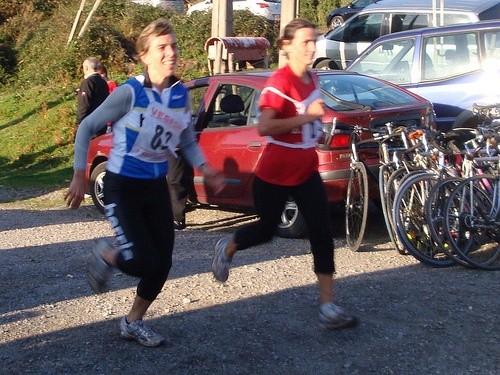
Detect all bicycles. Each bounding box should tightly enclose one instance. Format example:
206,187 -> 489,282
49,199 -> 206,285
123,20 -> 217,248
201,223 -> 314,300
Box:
330,101 -> 499,274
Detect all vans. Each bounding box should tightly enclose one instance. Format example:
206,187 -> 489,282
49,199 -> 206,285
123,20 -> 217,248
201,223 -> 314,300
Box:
309,1 -> 499,66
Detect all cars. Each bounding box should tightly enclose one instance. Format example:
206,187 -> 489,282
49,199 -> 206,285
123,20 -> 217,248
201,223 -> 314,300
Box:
185,0 -> 280,22
345,19 -> 500,121
81,72 -> 437,239
325,1 -> 377,28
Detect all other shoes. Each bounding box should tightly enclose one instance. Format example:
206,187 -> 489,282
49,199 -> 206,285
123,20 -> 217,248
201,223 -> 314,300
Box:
174,221 -> 186,230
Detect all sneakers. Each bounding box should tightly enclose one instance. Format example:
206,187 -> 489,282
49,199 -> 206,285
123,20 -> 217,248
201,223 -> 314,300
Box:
319,303 -> 361,332
211,235 -> 234,282
87,239 -> 112,293
119,315 -> 165,347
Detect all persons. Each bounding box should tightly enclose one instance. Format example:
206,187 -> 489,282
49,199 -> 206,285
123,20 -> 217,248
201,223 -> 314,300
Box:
212,18 -> 358,330
76,56 -> 118,141
63,19 -> 228,348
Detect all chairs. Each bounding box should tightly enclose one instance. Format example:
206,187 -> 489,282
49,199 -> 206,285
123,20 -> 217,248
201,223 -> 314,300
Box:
445,49 -> 459,61
213,95 -> 248,125
426,54 -> 435,77
